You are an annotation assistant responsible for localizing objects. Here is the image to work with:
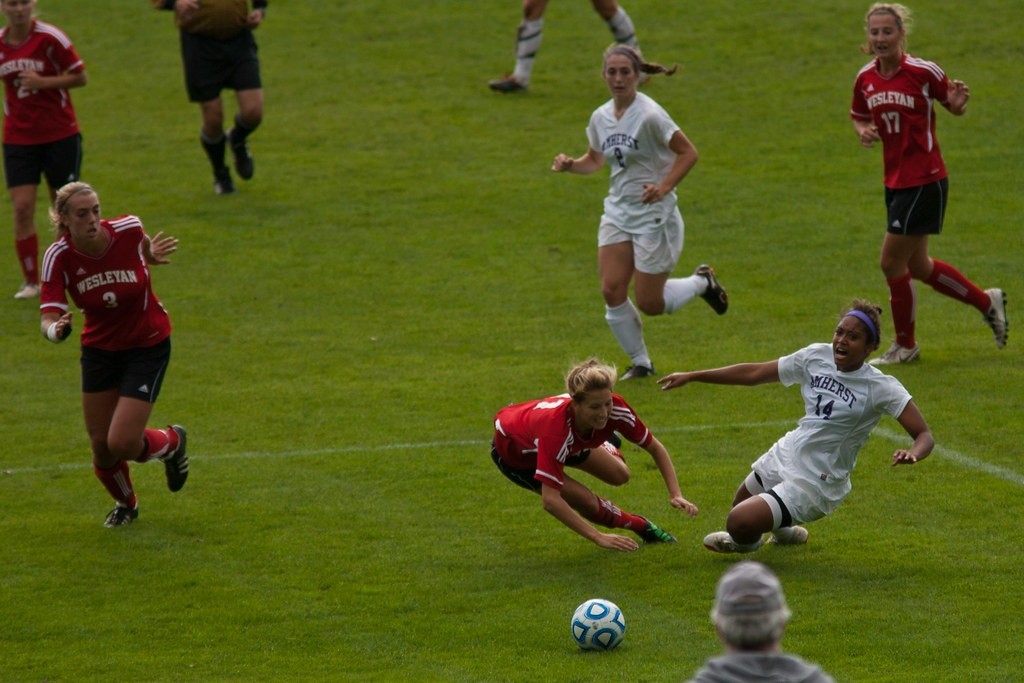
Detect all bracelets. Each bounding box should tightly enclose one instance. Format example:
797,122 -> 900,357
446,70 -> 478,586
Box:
47,322 -> 61,345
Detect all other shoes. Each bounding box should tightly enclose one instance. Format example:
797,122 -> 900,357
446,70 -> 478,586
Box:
226,128 -> 254,180
488,75 -> 531,95
213,170 -> 236,195
13,281 -> 42,300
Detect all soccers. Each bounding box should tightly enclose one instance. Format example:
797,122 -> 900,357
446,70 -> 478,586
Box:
570,598 -> 625,652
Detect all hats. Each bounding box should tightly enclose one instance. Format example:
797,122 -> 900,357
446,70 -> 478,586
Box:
710,561 -> 790,641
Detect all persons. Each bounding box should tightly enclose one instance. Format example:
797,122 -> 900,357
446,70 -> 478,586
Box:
491,358 -> 698,553
850,3 -> 1010,365
689,560 -> 836,683
655,300 -> 935,554
550,43 -> 729,382
0,0 -> 87,298
150,0 -> 269,195
489,0 -> 649,93
39,183 -> 189,530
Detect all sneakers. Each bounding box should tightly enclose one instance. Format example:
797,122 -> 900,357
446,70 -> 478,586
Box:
868,340 -> 920,365
703,531 -> 762,553
696,264 -> 728,315
608,432 -> 623,449
764,526 -> 809,545
103,495 -> 139,529
631,514 -> 679,546
157,424 -> 189,492
984,288 -> 1009,349
618,361 -> 656,382
